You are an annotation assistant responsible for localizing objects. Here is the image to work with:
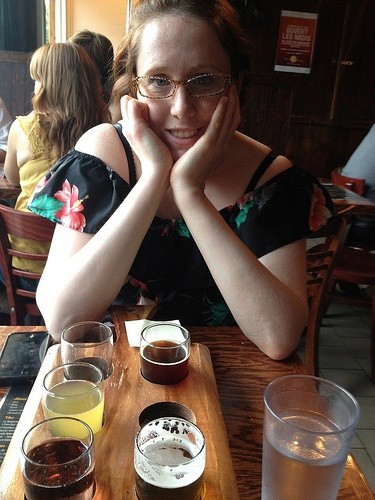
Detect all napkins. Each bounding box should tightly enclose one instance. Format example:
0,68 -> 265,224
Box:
124,319 -> 189,349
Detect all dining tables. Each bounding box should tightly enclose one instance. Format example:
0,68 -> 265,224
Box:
0,325 -> 375,500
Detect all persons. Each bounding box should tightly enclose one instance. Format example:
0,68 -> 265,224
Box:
0,30 -> 130,326
340,123 -> 375,297
26,0 -> 313,360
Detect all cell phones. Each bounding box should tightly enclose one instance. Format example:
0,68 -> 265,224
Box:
0,331 -> 51,384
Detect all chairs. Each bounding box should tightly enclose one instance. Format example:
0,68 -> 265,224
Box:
290,165 -> 375,394
0,203 -> 57,326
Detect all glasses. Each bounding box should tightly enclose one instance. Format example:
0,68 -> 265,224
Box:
132,73 -> 230,100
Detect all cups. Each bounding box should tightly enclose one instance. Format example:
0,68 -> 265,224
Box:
138,323 -> 191,384
60,320 -> 113,381
132,417 -> 208,500
262,376 -> 359,500
42,362 -> 106,440
19,415 -> 94,500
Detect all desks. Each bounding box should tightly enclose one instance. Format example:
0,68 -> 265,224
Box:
0,159 -> 375,336
